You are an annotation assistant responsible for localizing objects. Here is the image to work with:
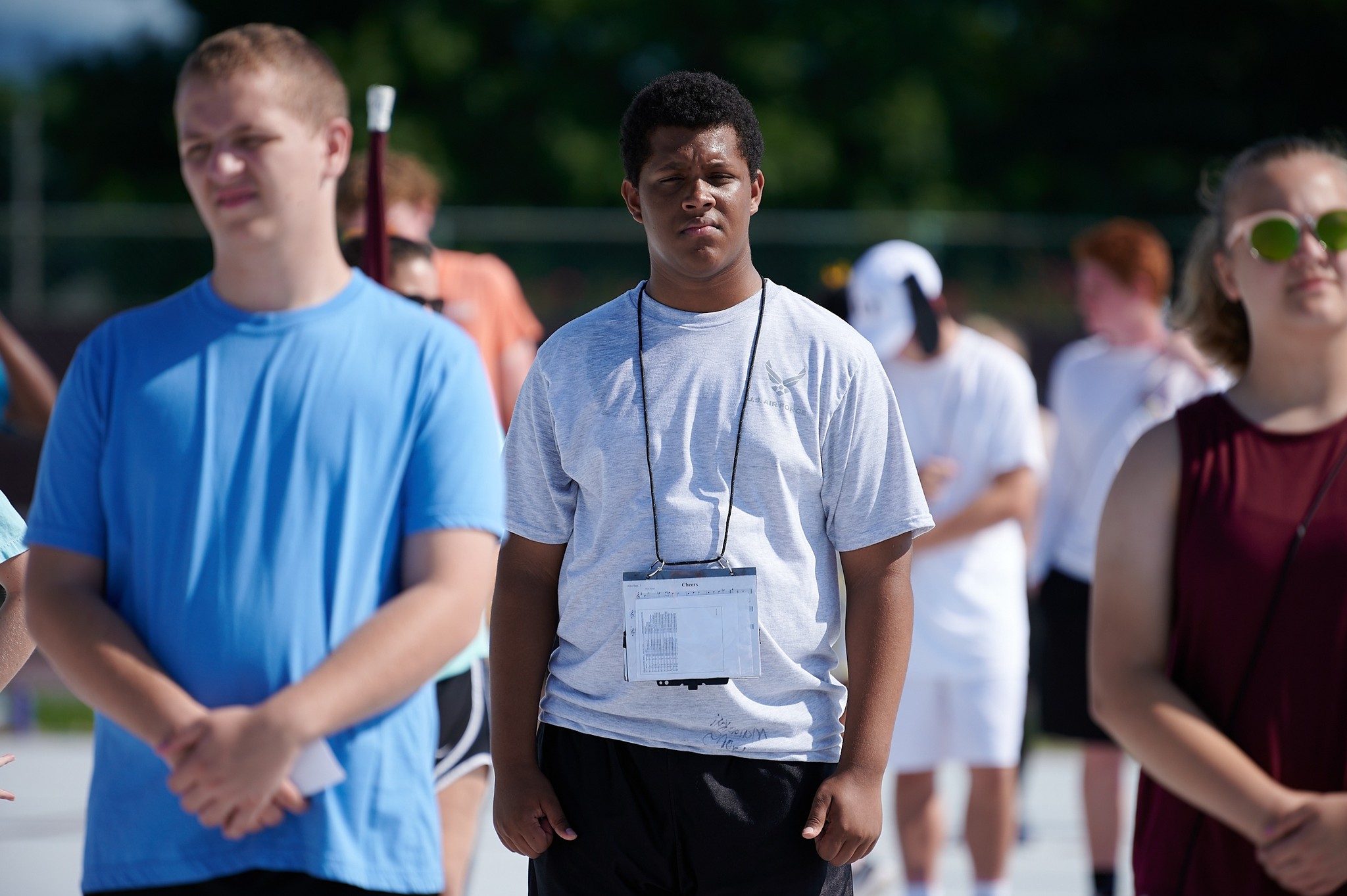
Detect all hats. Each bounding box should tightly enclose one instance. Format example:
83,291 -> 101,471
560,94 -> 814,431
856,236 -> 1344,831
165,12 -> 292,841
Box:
847,237 -> 944,358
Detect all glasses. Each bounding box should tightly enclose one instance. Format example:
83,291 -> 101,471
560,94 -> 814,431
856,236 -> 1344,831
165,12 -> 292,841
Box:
405,294 -> 445,316
1224,206 -> 1346,264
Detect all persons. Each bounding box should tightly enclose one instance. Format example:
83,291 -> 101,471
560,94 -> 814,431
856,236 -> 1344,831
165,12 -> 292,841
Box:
488,72 -> 937,896
22,24 -> 506,896
0,490 -> 38,692
840,230 -> 1229,896
1089,137 -> 1347,896
343,149 -> 543,896
0,313 -> 60,439
